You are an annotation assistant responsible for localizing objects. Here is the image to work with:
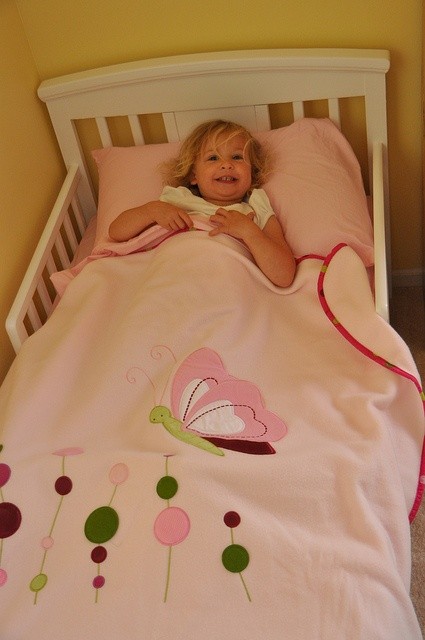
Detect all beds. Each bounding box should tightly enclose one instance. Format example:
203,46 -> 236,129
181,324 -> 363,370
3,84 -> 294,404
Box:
0,45 -> 425,636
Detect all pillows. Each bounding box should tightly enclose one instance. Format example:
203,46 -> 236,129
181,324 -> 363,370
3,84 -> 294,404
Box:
87,117 -> 375,266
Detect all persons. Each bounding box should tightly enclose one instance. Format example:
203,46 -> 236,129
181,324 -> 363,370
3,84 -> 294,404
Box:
108,120 -> 297,288
